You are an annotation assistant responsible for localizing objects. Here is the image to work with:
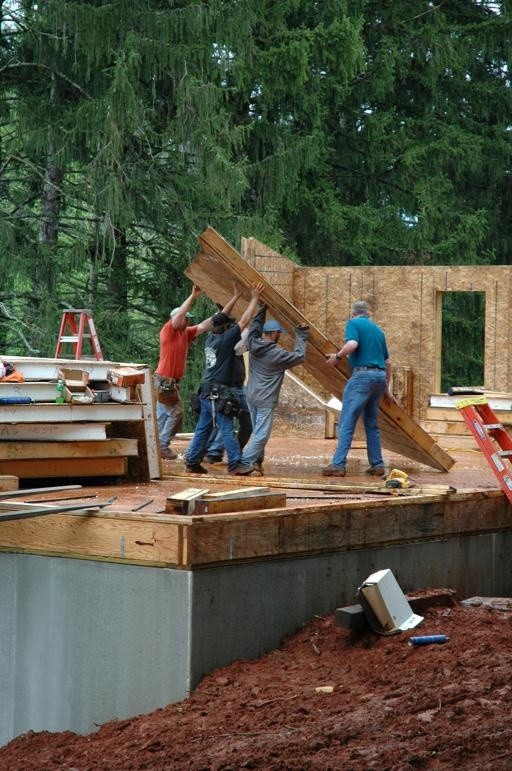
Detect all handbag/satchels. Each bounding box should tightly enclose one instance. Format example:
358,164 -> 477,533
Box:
158,379 -> 178,404
191,395 -> 200,410
219,397 -> 240,415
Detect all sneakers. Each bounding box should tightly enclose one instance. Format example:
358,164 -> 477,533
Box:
185,455 -> 262,477
366,466 -> 384,476
162,447 -> 177,459
323,463 -> 345,476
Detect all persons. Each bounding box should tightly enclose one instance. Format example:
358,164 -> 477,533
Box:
322,301 -> 390,476
154,282 -> 216,459
206,317 -> 254,463
186,282 -> 268,474
244,301 -> 310,463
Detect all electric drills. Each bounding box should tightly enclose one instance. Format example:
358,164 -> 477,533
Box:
381,469 -> 416,489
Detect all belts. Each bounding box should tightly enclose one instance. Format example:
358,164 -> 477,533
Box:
353,367 -> 384,371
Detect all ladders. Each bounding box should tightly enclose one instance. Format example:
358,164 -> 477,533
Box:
455,397 -> 512,504
55,309 -> 104,361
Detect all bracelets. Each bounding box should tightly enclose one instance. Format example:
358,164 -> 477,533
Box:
334,353 -> 342,359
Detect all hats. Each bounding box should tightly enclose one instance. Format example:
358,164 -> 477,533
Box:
263,321 -> 283,332
170,307 -> 195,317
211,313 -> 235,325
